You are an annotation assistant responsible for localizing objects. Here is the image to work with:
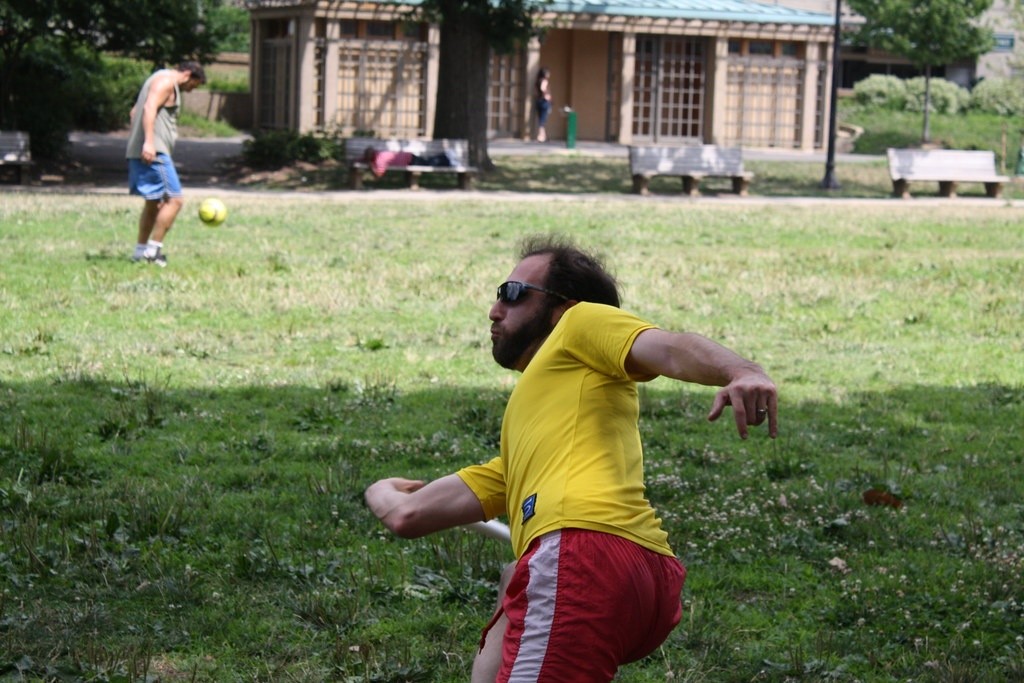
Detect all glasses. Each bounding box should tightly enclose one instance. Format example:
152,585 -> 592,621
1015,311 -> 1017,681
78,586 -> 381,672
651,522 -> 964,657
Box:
496,281 -> 568,302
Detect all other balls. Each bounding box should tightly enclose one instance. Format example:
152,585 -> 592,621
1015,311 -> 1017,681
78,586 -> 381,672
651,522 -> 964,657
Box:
198,199 -> 227,227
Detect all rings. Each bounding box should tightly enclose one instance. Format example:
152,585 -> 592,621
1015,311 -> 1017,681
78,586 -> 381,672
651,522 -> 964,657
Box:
759,406 -> 768,413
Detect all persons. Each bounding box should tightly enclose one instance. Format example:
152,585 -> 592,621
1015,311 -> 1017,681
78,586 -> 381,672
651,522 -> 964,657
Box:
128,63 -> 205,267
535,67 -> 552,143
353,147 -> 460,177
364,243 -> 777,683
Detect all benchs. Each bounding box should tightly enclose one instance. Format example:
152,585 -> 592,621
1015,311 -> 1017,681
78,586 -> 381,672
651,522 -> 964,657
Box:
627,144 -> 755,198
342,135 -> 478,192
0,131 -> 34,185
886,147 -> 1012,199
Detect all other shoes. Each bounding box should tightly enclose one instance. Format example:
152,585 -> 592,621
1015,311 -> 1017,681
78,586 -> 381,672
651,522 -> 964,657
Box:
134,249 -> 166,267
